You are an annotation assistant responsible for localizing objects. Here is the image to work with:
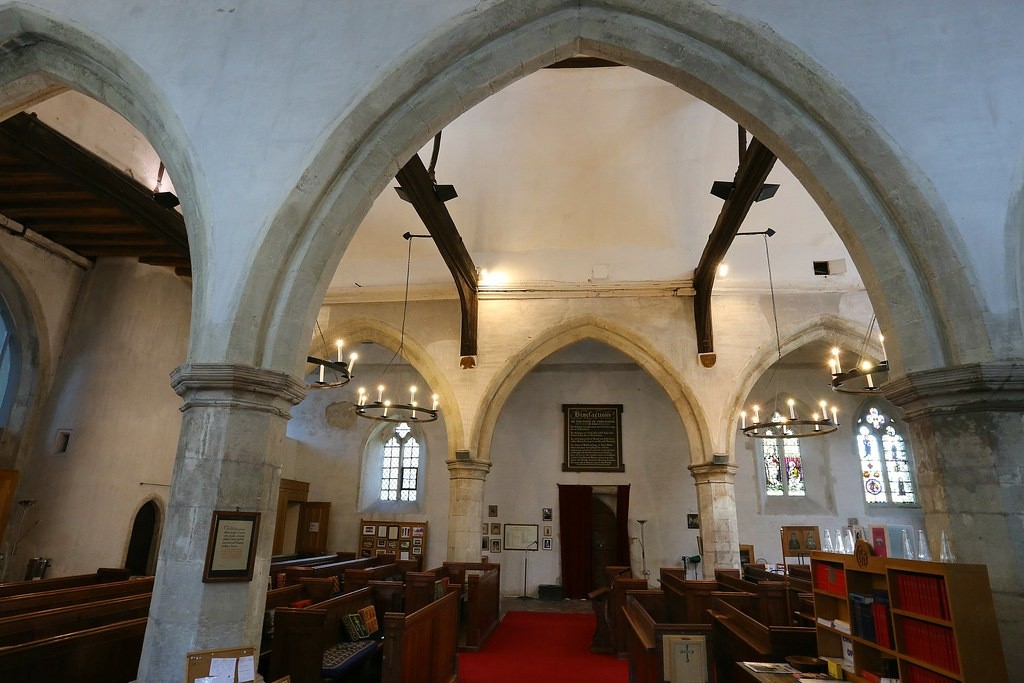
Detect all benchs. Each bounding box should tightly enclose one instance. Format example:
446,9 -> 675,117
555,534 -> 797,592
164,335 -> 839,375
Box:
607,564 -> 817,683
1,552 -> 500,683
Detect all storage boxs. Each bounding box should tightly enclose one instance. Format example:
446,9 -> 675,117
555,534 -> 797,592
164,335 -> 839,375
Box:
828,659 -> 842,679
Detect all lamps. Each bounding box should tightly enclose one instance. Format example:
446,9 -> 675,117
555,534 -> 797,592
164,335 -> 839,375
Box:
300,320 -> 358,390
828,315 -> 888,395
630,519 -> 650,580
356,232 -> 438,423
731,227 -> 840,438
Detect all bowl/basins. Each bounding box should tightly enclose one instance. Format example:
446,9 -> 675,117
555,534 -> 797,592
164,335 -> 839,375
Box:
785,655 -> 827,673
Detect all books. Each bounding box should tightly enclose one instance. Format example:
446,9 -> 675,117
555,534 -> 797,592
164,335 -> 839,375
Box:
743,617 -> 900,683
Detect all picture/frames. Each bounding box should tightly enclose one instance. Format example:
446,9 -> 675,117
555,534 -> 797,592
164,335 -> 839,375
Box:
687,514 -> 700,529
362,525 -> 423,561
697,536 -> 703,555
544,526 -> 552,536
542,508 -> 552,521
542,538 -> 552,550
482,505 -> 501,553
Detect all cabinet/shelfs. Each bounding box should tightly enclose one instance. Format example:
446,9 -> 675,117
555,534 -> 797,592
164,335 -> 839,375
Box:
810,550 -> 1009,683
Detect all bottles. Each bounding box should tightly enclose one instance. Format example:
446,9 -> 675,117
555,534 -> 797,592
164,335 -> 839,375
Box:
823,530 -> 834,553
788,532 -> 800,552
900,529 -> 916,560
835,530 -> 846,554
844,530 -> 855,555
917,529 -> 933,562
805,531 -> 816,550
939,530 -> 955,563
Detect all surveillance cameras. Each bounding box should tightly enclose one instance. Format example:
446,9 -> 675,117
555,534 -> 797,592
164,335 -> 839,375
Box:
812,258 -> 847,276
